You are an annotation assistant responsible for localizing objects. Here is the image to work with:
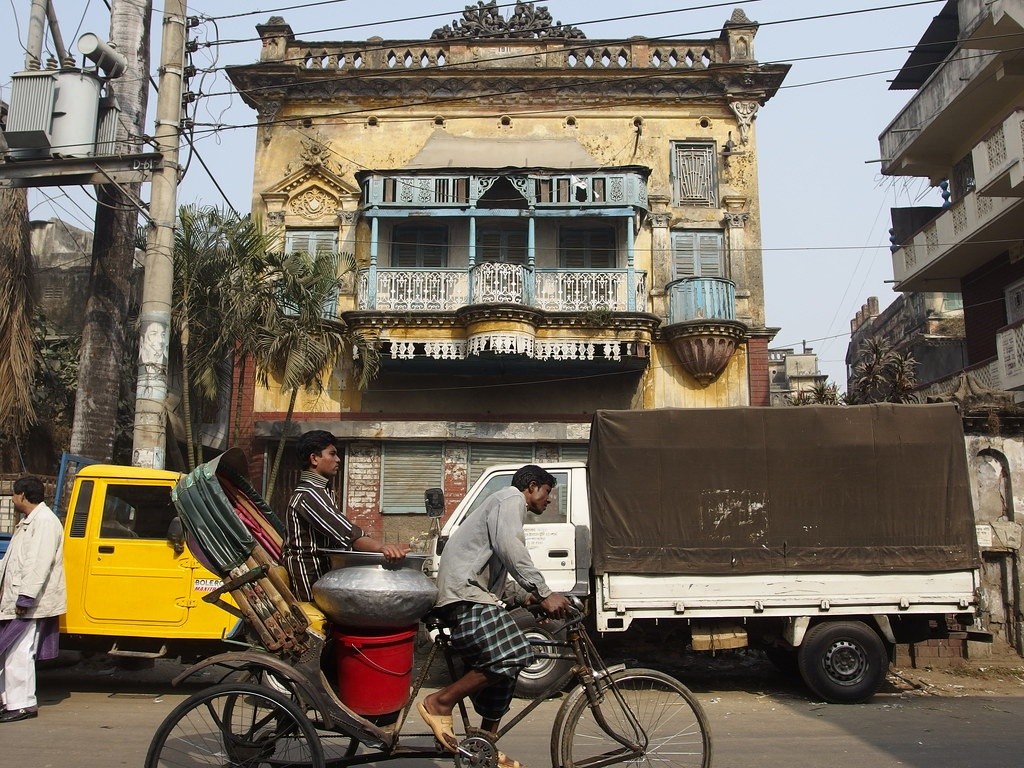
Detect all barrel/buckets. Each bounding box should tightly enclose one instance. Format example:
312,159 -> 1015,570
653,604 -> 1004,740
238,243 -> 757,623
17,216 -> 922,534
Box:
331,627 -> 414,717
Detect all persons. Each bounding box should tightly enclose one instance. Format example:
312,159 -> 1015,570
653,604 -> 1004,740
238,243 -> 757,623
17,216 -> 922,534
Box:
419,465 -> 572,768
0,475 -> 66,722
281,430 -> 410,603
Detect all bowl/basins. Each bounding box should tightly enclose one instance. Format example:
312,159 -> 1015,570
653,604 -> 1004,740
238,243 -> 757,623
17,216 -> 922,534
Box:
312,548 -> 437,635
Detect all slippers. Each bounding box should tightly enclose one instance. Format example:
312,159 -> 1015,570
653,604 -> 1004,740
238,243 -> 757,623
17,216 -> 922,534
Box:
417,702 -> 459,753
485,751 -> 520,768
0,705 -> 38,723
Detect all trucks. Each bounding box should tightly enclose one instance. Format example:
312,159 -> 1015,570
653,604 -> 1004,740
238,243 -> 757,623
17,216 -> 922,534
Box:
0,452 -> 298,708
424,403 -> 994,706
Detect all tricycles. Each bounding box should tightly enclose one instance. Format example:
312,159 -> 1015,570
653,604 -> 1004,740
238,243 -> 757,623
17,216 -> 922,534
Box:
144,444 -> 714,768
